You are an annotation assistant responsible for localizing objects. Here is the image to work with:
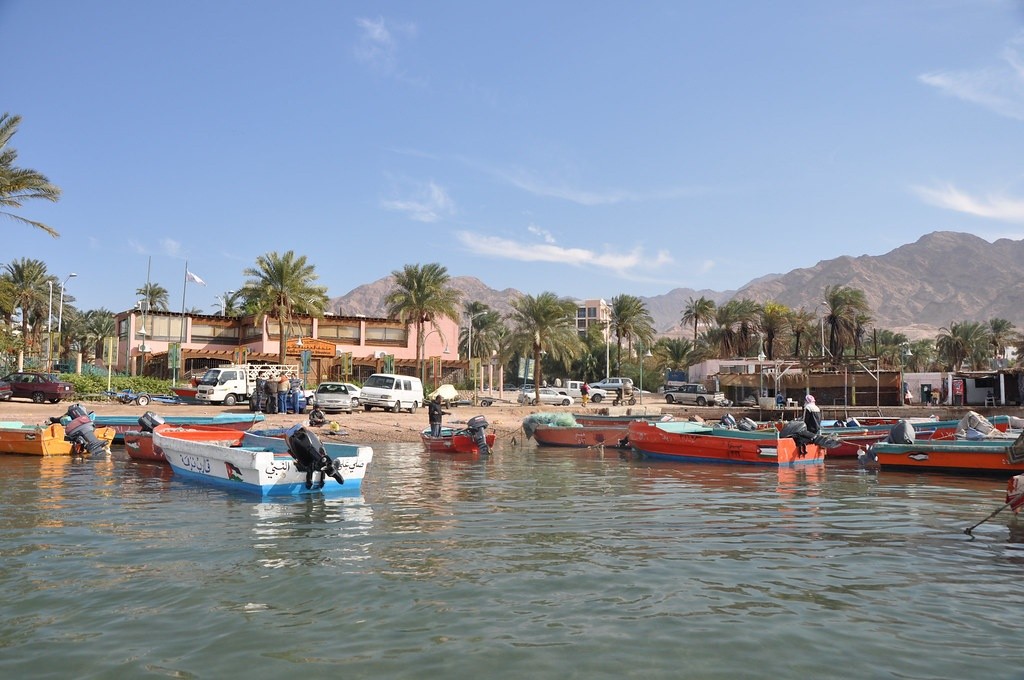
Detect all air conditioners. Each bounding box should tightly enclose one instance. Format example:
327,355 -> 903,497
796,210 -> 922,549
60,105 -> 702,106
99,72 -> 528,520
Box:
138,344 -> 150,352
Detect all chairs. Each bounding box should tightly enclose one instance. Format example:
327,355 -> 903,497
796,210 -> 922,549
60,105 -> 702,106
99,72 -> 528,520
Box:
22,378 -> 28,382
984,393 -> 996,406
33,379 -> 38,383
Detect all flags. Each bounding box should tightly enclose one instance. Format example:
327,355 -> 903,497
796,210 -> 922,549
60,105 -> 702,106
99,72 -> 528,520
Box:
186,271 -> 207,288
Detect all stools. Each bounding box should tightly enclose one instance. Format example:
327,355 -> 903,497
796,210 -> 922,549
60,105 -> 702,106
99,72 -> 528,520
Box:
775,403 -> 784,410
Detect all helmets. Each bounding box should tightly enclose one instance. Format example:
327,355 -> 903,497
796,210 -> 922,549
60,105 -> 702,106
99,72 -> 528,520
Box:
313,403 -> 320,409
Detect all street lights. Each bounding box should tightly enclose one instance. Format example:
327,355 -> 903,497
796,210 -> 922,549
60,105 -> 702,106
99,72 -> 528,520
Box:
639,336 -> 653,405
900,342 -> 914,406
214,291 -> 235,316
285,326 -> 305,364
47,273 -> 77,332
138,310 -> 147,377
605,303 -> 617,377
468,311 -> 488,361
422,330 -> 450,387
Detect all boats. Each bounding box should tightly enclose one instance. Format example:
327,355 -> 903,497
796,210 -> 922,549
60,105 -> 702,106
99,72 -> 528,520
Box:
1,411 -> 375,499
529,413 -> 1024,477
421,424 -> 496,451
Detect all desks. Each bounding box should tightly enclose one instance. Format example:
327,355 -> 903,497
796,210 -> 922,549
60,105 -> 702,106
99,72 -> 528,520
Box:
786,401 -> 798,408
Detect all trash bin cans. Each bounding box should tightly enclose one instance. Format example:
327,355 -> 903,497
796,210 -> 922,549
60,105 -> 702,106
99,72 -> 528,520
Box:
921,384 -> 931,402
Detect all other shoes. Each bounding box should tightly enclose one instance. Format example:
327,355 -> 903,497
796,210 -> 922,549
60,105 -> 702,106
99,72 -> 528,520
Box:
254,410 -> 300,415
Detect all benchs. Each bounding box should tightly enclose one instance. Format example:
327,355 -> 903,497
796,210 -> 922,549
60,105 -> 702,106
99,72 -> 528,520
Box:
233,446 -> 271,451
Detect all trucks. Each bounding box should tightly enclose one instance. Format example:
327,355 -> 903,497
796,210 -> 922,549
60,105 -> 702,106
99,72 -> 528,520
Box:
194,362 -> 299,406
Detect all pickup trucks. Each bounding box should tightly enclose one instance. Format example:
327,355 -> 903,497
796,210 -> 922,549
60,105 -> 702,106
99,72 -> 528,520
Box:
663,383 -> 725,407
550,381 -> 607,402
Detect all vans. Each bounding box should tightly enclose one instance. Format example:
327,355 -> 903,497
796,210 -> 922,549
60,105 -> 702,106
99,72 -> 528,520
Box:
360,373 -> 424,414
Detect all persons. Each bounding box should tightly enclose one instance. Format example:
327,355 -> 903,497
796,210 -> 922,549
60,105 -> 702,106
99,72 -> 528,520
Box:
428,395 -> 452,437
255,371 -> 302,414
309,404 -> 325,427
804,395 -> 821,434
581,381 -> 591,407
776,391 -> 787,409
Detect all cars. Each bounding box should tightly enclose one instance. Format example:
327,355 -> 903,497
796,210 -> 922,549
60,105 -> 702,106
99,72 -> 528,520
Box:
249,382 -> 361,414
0,373 -> 75,403
589,377 -> 633,394
518,389 -> 574,406
